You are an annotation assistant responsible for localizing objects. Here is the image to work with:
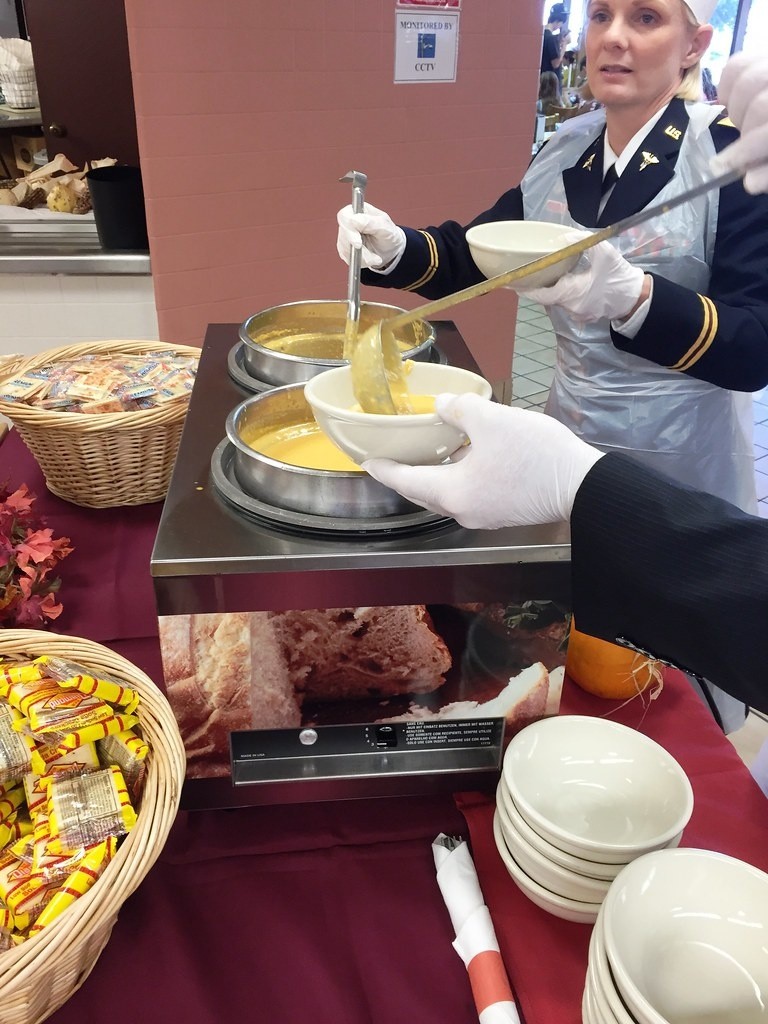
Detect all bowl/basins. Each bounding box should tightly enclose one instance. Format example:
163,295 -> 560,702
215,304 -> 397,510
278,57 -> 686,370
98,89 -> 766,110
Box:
304,360 -> 492,465
582,847 -> 768,1024
493,714 -> 695,923
466,219 -> 585,290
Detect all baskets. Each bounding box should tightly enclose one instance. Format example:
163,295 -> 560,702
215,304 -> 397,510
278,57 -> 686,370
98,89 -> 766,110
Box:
0,628 -> 187,1024
0,339 -> 203,509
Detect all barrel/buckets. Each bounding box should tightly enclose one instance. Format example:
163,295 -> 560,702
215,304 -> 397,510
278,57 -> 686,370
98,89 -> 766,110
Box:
85,165 -> 149,250
226,380 -> 420,518
238,300 -> 435,386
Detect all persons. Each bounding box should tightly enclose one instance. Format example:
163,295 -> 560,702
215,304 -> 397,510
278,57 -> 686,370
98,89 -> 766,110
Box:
338,2 -> 767,516
360,51 -> 767,718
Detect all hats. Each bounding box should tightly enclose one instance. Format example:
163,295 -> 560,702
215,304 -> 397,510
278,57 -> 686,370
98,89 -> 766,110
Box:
683,0 -> 719,26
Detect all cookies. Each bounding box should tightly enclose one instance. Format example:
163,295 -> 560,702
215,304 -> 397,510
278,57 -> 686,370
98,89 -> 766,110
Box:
0,656 -> 149,952
0,351 -> 200,413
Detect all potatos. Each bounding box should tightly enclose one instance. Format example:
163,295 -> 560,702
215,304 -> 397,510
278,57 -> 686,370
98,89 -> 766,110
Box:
0,189 -> 18,207
47,186 -> 78,214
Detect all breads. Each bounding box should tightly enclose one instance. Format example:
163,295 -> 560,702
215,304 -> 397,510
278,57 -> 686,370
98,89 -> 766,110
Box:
158,604 -> 564,779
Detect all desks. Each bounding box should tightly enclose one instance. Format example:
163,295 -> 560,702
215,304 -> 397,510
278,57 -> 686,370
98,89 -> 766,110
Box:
0,424 -> 768,1024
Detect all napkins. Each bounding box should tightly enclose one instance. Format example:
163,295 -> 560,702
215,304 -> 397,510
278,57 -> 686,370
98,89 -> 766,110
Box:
431,833 -> 521,1024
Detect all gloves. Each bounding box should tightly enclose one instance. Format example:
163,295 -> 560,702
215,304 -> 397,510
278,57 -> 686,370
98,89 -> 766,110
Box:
337,202 -> 407,269
514,231 -> 645,324
359,393 -> 607,529
708,43 -> 768,195
